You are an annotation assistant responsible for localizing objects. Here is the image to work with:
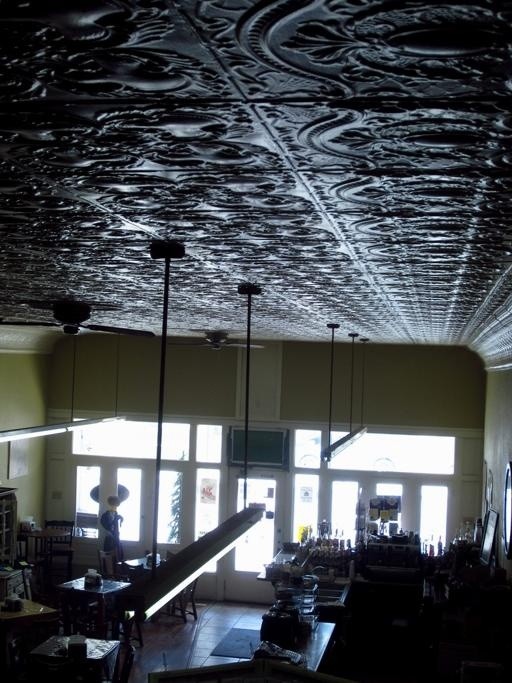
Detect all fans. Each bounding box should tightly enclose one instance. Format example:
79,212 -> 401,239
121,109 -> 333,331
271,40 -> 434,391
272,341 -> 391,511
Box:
167,332 -> 265,351
1,301 -> 156,337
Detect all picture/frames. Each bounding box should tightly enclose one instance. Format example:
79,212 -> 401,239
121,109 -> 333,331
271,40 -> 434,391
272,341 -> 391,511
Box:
478,509 -> 499,565
501,462 -> 511,560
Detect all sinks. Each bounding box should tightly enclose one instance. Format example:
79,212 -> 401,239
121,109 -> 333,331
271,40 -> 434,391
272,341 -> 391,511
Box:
316,596 -> 340,603
318,590 -> 344,599
317,580 -> 347,591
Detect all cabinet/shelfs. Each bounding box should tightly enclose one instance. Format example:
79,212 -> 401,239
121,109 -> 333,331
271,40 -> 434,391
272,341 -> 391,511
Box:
1,488 -> 17,564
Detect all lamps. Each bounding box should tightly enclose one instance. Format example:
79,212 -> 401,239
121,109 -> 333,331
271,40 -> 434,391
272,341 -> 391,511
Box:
125,241 -> 263,621
321,324 -> 368,462
1,338 -> 126,441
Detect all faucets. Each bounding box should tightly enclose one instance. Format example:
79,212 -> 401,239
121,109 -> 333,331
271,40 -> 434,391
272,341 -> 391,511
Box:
311,565 -> 324,572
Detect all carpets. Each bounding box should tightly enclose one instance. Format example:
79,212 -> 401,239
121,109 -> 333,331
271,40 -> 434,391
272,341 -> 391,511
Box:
210,628 -> 260,658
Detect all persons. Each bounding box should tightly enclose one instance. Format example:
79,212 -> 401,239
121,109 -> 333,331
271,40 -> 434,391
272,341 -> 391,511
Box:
101,495 -> 124,561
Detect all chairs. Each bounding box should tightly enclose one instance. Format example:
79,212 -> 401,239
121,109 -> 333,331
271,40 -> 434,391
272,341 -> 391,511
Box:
17,519 -> 75,580
9,546 -> 198,683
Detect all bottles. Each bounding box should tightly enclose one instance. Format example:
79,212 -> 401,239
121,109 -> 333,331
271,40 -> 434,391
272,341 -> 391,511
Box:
422,535 -> 436,557
437,535 -> 443,557
316,528 -> 351,550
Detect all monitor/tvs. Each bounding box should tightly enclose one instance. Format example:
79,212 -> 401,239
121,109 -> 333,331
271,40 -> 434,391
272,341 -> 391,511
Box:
226,426 -> 292,474
